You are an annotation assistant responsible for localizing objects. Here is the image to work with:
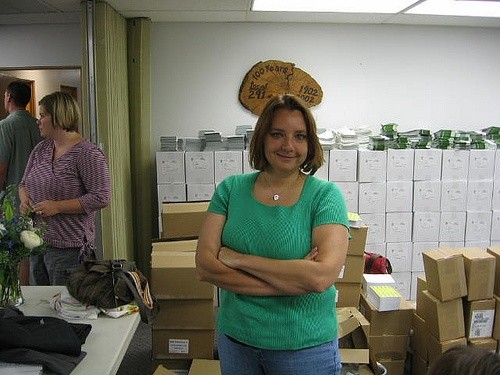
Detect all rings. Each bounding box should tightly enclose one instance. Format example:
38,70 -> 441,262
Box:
40,210 -> 43,214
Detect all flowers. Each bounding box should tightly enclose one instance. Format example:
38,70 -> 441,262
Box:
1,184 -> 50,269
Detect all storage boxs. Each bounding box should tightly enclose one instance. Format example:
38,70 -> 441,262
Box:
148,147 -> 500,375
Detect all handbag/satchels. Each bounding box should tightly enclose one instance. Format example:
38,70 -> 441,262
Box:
66,259 -> 161,325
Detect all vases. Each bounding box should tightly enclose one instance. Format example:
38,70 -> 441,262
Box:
1,261 -> 25,308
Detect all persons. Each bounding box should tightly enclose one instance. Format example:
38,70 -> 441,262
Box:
0,81 -> 45,286
428,345 -> 500,375
18,92 -> 111,286
195,94 -> 352,375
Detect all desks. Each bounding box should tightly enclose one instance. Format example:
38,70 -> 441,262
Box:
0,286 -> 142,375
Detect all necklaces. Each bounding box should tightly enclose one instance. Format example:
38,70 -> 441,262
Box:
262,175 -> 301,202
